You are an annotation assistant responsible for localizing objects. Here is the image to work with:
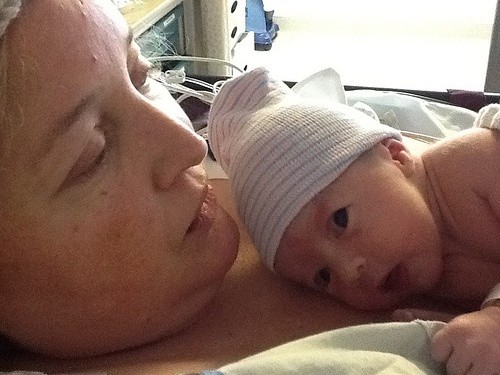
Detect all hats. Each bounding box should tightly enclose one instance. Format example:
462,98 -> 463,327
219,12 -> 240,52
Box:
207,65 -> 403,275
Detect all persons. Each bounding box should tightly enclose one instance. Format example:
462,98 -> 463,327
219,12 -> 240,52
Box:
1,0 -> 499,375
203,65 -> 500,375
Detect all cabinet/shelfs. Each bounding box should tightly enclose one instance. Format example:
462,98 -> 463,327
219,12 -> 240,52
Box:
115,0 -> 257,94
